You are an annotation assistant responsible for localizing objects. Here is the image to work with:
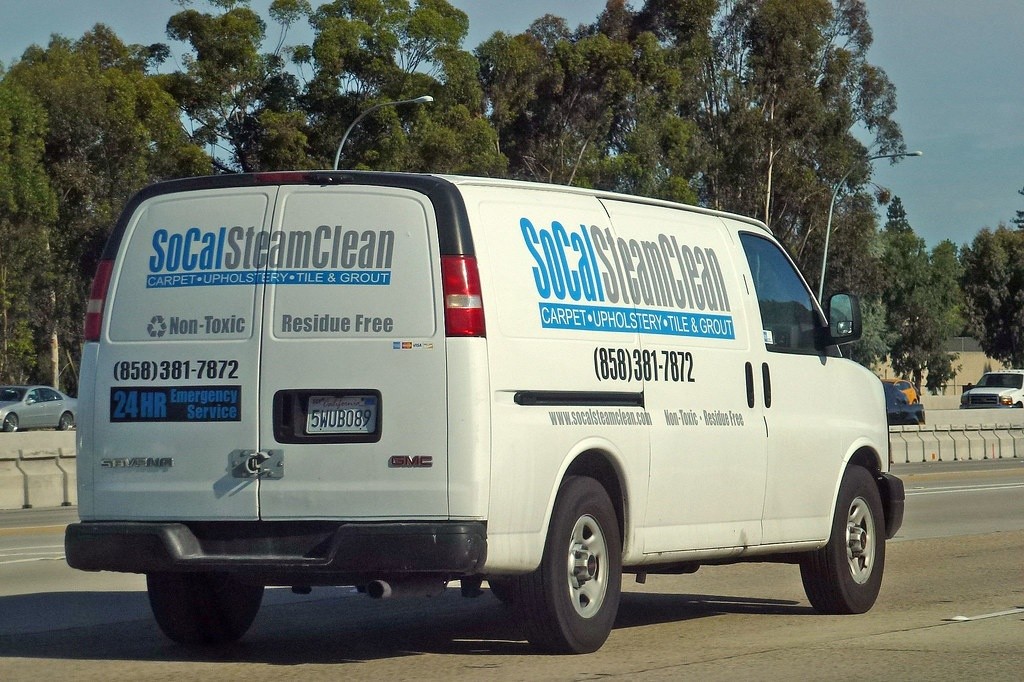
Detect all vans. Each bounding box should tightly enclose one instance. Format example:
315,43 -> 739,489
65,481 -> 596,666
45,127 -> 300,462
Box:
62,169 -> 905,660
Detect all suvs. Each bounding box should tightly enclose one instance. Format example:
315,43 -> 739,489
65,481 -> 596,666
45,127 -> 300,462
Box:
959,369 -> 1024,408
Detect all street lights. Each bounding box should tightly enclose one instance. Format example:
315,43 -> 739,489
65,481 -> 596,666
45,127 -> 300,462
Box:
817,151 -> 924,304
333,95 -> 433,171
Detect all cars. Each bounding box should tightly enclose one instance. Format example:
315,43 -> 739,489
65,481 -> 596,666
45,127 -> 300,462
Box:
882,383 -> 926,425
879,377 -> 919,405
0,384 -> 79,434
